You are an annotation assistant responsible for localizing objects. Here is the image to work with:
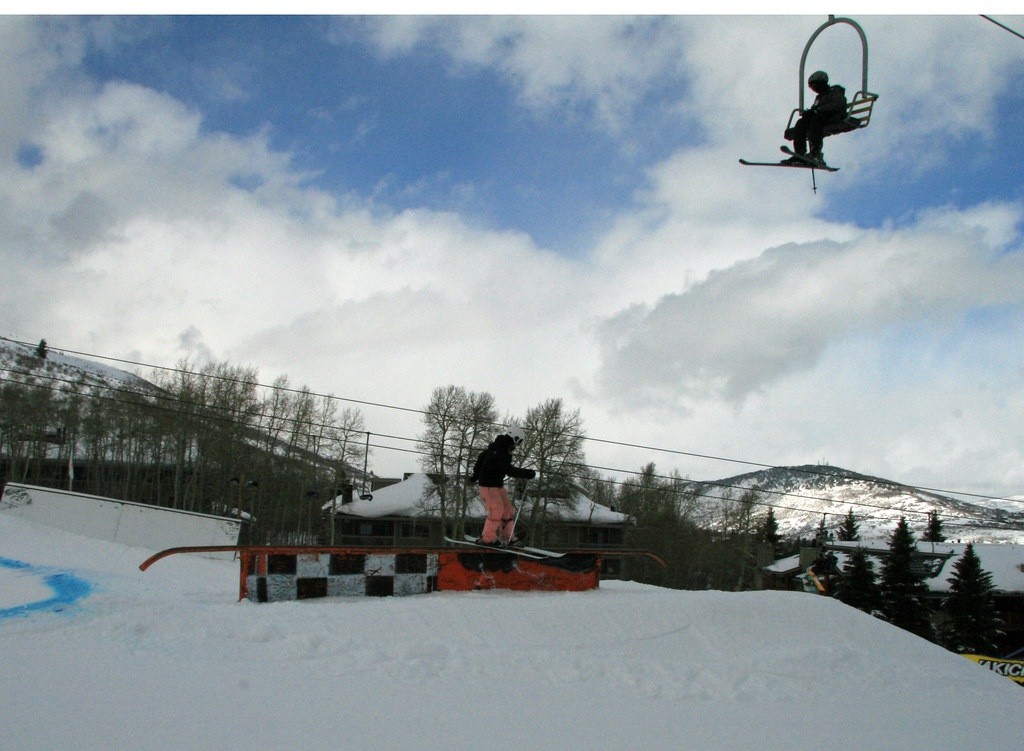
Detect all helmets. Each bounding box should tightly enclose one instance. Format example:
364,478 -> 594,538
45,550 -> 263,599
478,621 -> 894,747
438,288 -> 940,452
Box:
808,71 -> 829,83
506,425 -> 526,442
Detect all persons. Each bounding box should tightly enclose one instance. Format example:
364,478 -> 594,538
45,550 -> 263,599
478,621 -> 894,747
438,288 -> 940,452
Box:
780,71 -> 847,165
467,426 -> 536,547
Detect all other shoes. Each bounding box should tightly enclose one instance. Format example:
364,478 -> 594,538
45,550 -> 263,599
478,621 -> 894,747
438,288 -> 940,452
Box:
505,534 -> 522,547
781,153 -> 808,164
802,153 -> 828,168
476,539 -> 503,547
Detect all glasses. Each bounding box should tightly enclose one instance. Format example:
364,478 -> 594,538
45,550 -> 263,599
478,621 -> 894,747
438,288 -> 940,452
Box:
514,435 -> 523,444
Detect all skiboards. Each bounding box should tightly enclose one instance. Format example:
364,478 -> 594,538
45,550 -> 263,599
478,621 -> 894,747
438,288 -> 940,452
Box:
738,145 -> 840,172
445,533 -> 570,560
